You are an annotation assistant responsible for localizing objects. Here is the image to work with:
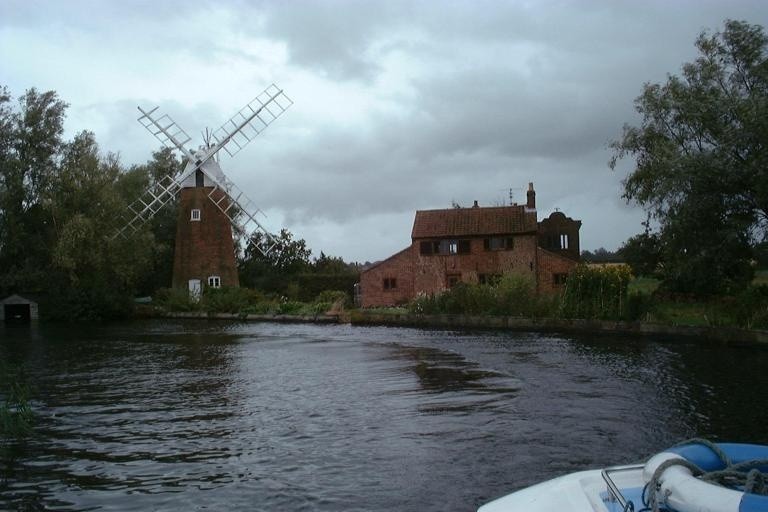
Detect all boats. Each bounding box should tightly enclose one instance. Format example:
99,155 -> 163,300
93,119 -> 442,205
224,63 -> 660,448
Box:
476,462 -> 768,512
